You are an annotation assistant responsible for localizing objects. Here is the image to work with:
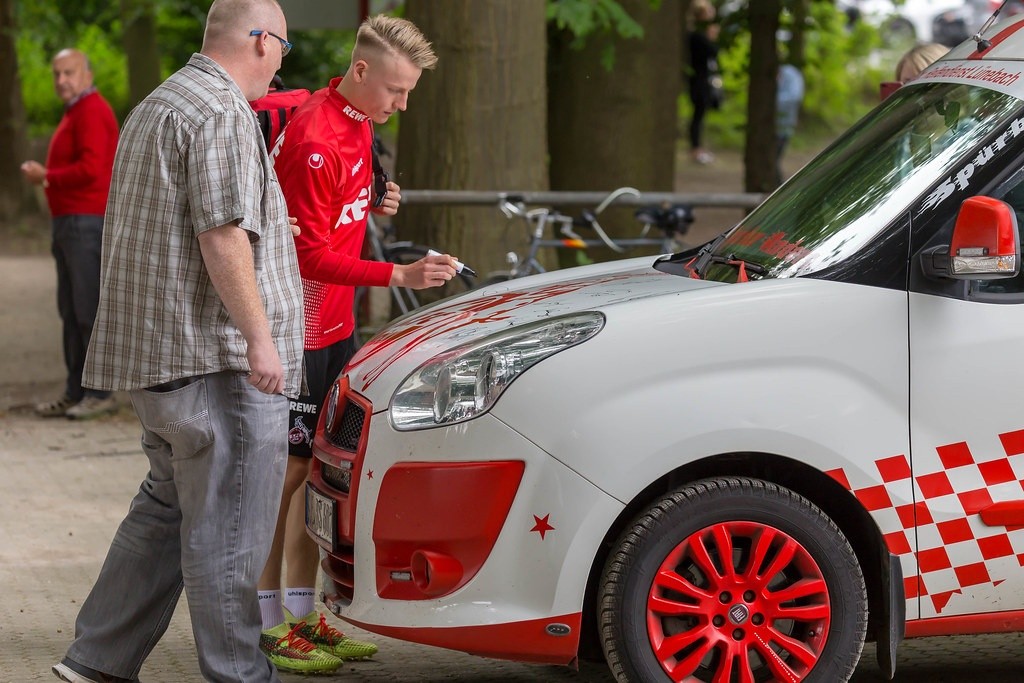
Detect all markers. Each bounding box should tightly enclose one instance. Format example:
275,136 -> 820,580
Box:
427,249 -> 478,278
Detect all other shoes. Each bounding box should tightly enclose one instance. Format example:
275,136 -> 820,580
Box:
691,147 -> 713,163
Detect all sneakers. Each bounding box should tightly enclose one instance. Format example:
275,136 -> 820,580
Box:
257,621 -> 344,675
34,393 -> 78,417
281,605 -> 377,660
65,393 -> 118,420
53,657 -> 142,683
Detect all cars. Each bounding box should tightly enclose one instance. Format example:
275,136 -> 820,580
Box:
305,0 -> 1024,683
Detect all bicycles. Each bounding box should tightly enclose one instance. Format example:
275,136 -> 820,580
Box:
350,212 -> 483,353
473,186 -> 702,285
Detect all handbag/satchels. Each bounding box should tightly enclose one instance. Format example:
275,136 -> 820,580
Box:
708,59 -> 721,110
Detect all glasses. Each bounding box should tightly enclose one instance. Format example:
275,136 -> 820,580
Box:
250,31 -> 292,57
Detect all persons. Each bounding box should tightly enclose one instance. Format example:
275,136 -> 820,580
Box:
52,0 -> 305,683
774,43 -> 806,186
257,14 -> 458,675
20,49 -> 120,421
896,41 -> 979,178
681,0 -> 724,165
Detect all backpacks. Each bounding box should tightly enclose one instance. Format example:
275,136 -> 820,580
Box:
246,74 -> 390,209
